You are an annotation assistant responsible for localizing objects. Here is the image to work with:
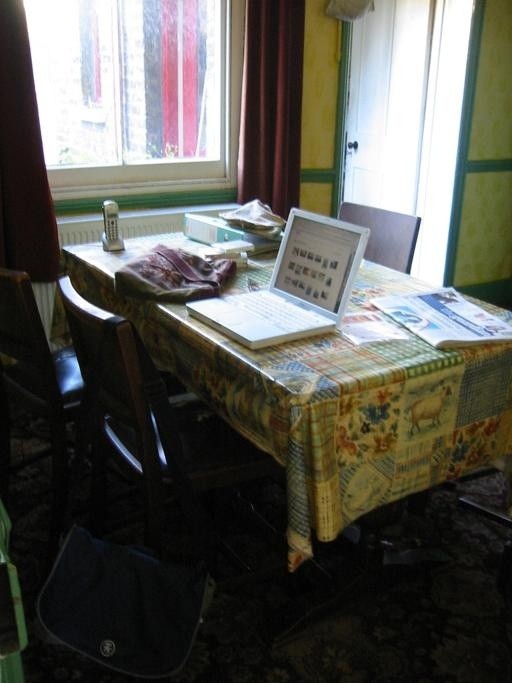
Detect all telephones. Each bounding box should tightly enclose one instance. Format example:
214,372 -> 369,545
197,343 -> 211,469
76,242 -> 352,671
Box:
101,200 -> 126,252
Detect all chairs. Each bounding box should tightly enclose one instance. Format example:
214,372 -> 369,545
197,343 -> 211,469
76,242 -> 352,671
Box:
336,202 -> 421,274
46,266 -> 305,678
1,267 -> 103,502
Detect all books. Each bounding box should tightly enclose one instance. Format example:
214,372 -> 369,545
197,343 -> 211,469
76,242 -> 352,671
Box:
199,239 -> 254,270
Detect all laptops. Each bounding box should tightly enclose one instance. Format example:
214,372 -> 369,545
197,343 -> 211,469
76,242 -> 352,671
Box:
184,207 -> 371,350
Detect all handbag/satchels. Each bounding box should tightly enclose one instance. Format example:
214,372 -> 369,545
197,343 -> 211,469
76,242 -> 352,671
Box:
113,244 -> 236,304
30,524 -> 217,681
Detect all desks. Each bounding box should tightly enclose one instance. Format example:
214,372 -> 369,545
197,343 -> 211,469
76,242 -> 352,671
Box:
54,197 -> 512,574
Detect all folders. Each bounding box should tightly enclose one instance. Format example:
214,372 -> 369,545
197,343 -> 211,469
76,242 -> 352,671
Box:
182,213 -> 283,256
